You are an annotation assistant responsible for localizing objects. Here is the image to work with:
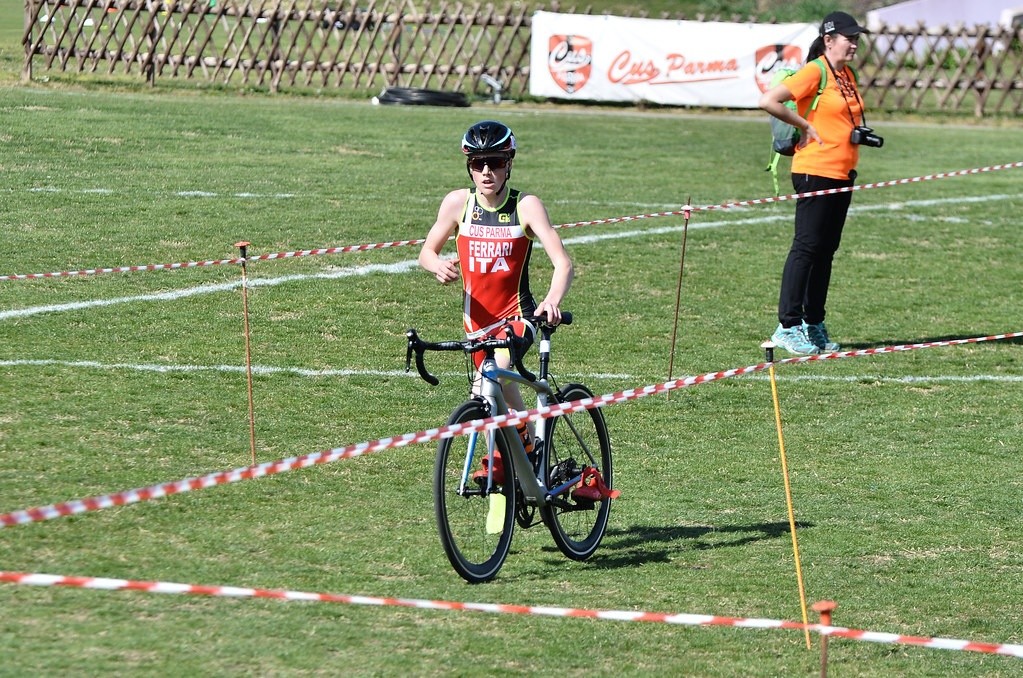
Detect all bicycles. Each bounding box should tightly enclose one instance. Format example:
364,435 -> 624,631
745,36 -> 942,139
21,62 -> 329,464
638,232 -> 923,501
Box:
405,311 -> 613,584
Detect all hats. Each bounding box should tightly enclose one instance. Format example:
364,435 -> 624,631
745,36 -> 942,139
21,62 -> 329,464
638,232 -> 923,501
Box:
819,11 -> 870,36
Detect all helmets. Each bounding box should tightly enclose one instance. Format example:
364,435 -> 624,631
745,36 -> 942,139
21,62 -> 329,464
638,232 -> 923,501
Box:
461,121 -> 516,158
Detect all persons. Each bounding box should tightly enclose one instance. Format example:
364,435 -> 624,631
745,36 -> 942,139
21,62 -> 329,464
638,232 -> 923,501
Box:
759,11 -> 872,357
417,121 -> 575,532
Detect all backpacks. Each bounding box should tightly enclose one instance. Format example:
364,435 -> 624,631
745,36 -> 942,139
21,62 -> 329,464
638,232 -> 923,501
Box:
765,59 -> 860,156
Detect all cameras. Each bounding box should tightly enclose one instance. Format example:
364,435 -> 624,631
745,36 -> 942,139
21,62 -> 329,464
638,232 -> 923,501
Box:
852,125 -> 884,148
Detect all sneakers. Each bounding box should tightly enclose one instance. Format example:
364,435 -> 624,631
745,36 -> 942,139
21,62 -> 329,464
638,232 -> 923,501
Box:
472,450 -> 504,483
771,323 -> 821,356
802,319 -> 840,353
571,466 -> 620,501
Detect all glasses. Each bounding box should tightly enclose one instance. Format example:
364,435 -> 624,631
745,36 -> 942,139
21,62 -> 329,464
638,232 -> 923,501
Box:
467,156 -> 514,172
